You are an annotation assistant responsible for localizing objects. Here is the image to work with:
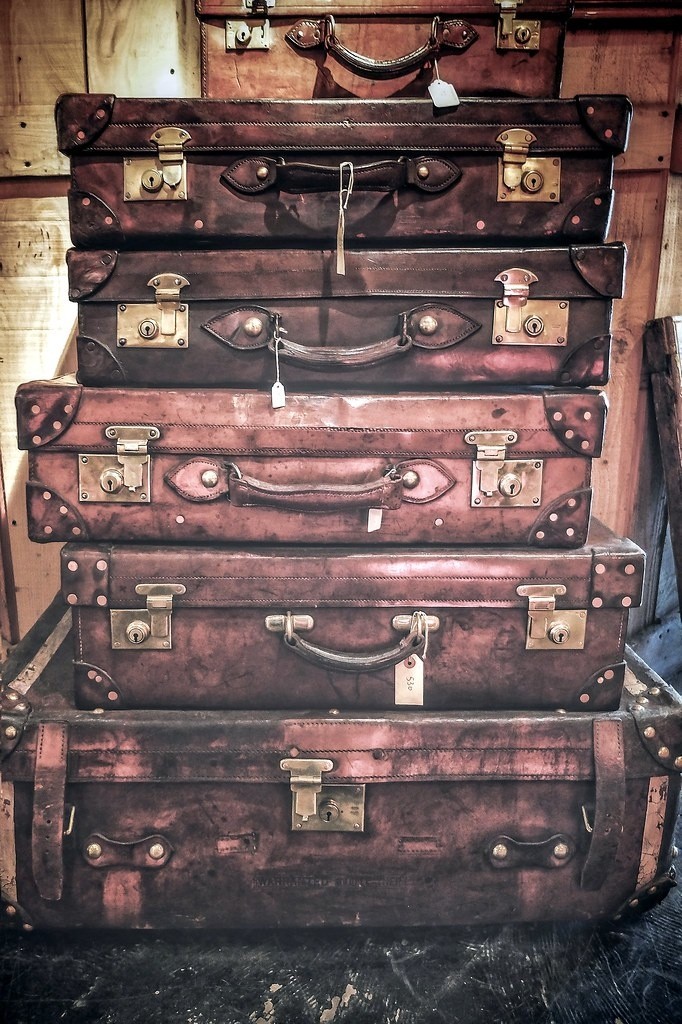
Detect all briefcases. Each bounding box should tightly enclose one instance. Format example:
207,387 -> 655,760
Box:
0,1 -> 682,930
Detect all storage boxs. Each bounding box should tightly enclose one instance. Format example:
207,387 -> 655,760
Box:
0,589 -> 682,930
